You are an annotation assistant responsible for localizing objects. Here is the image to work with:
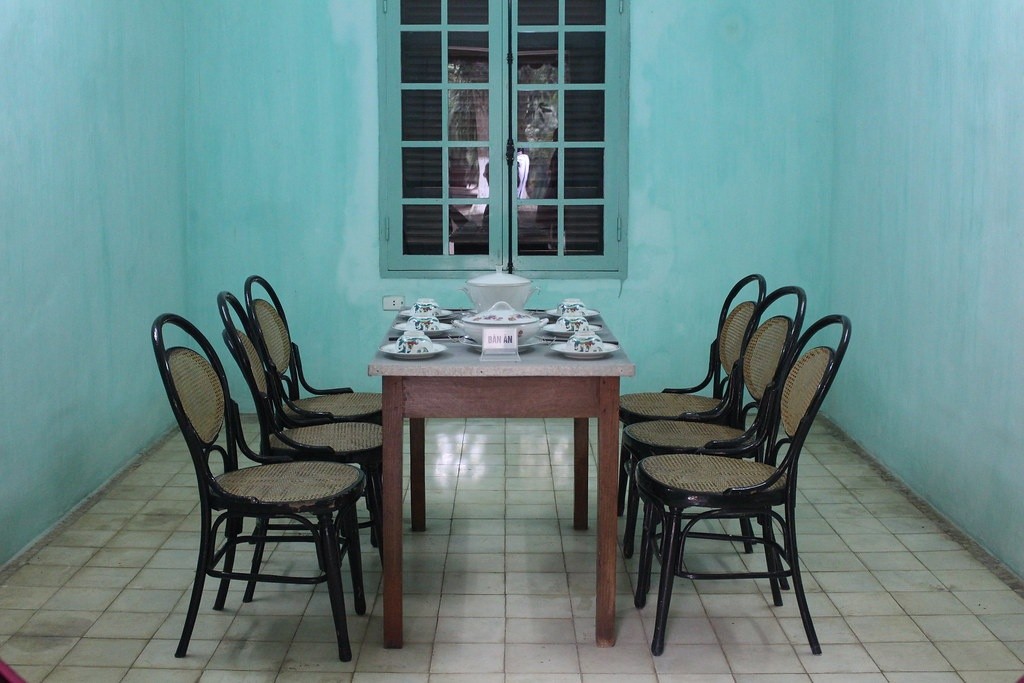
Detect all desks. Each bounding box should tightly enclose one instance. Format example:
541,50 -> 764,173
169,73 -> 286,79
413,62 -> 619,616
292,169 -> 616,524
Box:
368,308 -> 634,649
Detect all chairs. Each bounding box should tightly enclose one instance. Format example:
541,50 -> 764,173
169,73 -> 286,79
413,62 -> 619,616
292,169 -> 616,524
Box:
151,274 -> 383,662
617,272 -> 852,657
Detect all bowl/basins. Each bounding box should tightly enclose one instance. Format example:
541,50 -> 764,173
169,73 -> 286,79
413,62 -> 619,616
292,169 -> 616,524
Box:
411,297 -> 440,317
555,316 -> 590,332
395,330 -> 433,353
557,298 -> 587,316
567,331 -> 603,352
406,315 -> 440,330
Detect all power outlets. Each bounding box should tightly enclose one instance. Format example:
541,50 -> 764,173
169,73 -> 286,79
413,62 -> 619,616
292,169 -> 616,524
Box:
383,294 -> 405,311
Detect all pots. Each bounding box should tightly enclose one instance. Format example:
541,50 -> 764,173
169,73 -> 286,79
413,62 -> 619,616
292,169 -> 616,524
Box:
457,265 -> 539,315
453,301 -> 549,343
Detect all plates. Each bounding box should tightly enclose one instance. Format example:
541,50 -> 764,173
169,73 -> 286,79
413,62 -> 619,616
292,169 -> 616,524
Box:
457,335 -> 542,353
551,342 -> 619,359
390,321 -> 455,338
378,343 -> 448,360
545,308 -> 601,320
543,324 -> 603,338
398,309 -> 453,317
